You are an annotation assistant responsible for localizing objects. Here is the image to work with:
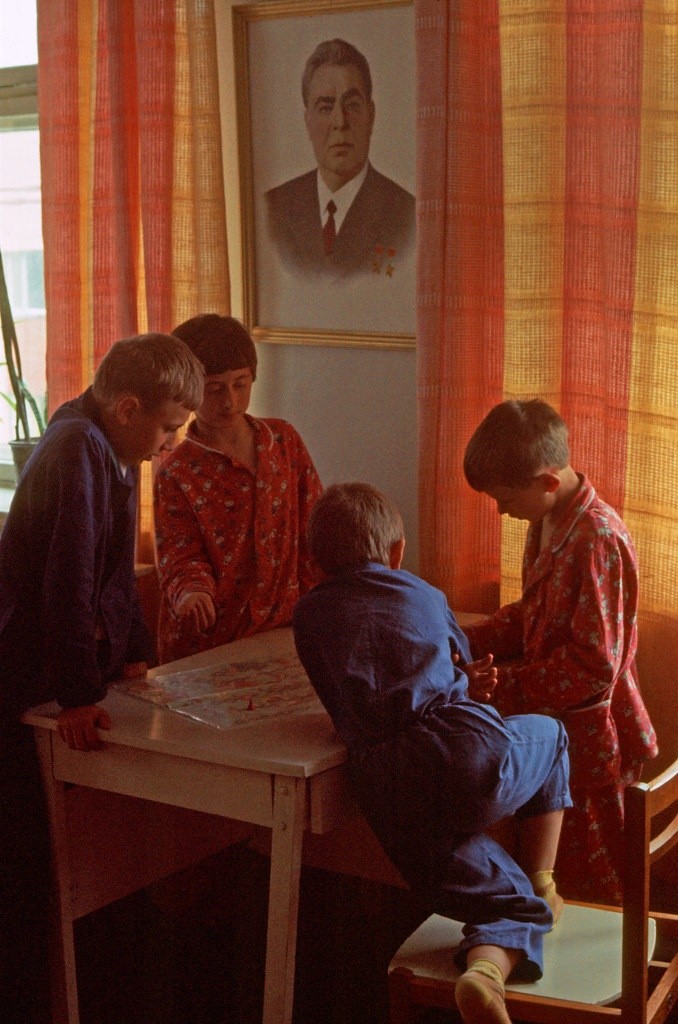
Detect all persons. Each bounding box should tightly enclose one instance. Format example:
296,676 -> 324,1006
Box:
288,482 -> 573,1022
453,396 -> 659,914
151,313 -> 328,667
3,332 -> 210,1021
260,37 -> 418,286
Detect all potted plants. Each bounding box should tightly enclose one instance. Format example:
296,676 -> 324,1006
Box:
2,251 -> 50,492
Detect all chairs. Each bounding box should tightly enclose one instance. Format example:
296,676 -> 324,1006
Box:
383,757 -> 678,1024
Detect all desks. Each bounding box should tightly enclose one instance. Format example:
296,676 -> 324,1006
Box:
20,607 -> 497,1023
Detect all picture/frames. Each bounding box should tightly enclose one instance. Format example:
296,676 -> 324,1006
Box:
231,2 -> 423,351
